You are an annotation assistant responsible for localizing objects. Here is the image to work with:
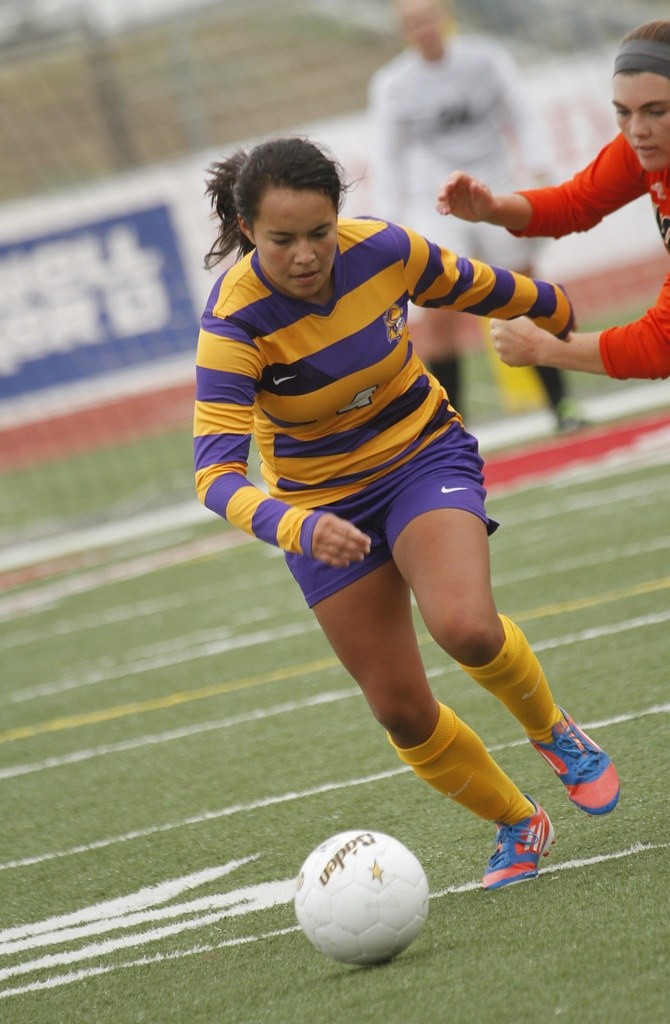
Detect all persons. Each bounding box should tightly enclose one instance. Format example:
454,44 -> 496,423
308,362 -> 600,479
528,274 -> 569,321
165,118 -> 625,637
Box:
193,138 -> 621,887
437,20 -> 670,381
366,0 -> 586,433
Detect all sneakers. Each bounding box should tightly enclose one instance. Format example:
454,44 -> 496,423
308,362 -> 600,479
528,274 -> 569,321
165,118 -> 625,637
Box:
528,705 -> 620,815
481,795 -> 555,892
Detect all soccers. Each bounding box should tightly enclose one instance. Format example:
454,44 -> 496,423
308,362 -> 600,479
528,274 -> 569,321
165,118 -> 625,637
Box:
291,830 -> 427,966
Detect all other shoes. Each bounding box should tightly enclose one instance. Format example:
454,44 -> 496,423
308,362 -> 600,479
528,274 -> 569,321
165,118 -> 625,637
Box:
556,398 -> 586,435
